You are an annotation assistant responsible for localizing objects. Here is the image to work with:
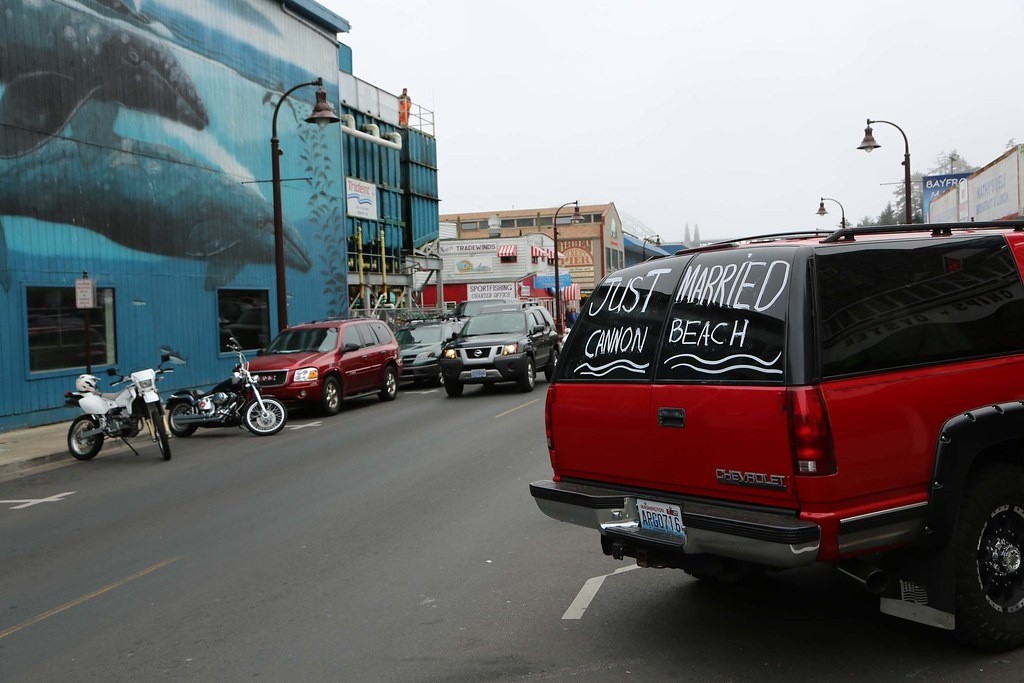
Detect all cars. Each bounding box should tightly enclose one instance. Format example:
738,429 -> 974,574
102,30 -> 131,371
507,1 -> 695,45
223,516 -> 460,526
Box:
222,304 -> 270,349
559,328 -> 572,354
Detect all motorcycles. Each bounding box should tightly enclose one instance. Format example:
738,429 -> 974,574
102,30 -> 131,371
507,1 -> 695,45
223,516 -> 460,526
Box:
162,336 -> 288,436
63,353 -> 176,462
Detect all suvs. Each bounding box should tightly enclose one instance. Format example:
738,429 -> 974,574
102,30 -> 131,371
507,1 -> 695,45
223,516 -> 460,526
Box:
394,315 -> 468,388
527,217 -> 1023,655
447,297 -> 522,317
437,302 -> 559,398
242,318 -> 405,417
27,319 -> 106,371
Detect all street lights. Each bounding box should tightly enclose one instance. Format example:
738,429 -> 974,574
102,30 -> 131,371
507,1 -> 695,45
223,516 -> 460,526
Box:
269,76 -> 341,334
858,118 -> 913,226
641,234 -> 663,263
555,200 -> 586,334
815,197 -> 846,229
